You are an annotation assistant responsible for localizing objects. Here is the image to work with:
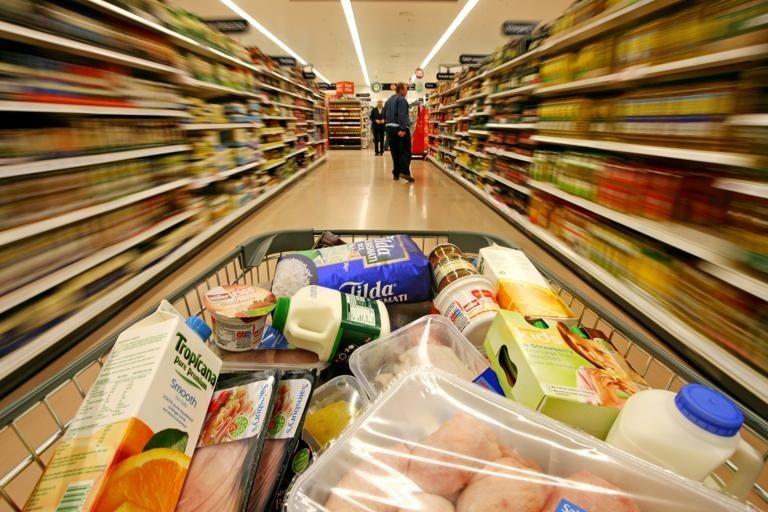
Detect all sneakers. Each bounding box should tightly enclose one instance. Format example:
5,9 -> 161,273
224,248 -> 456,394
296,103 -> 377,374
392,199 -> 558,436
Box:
375,152 -> 383,155
393,171 -> 415,182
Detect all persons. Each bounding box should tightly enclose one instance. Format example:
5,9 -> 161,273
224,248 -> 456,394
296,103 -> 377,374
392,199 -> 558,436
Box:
380,81 -> 416,184
369,98 -> 386,157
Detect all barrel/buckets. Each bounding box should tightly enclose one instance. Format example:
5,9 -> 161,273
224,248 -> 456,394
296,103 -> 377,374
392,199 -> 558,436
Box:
272,284 -> 391,364
607,385 -> 764,502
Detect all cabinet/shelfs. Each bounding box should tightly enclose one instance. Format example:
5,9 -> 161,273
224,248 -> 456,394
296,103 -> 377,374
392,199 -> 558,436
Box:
0,0 -> 768,419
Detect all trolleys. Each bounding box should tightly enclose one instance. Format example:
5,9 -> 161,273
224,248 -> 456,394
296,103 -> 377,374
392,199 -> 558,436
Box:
1,221 -> 768,512
